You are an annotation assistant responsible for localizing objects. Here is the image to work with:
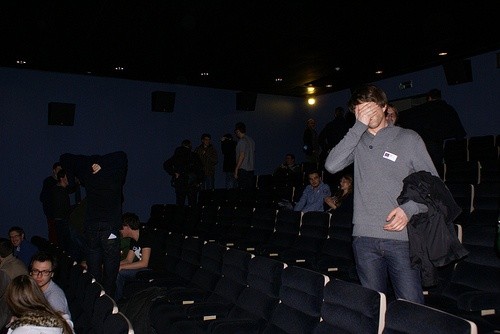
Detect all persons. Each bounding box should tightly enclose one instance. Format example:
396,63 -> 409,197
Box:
418,90 -> 462,166
324,85 -> 442,304
0,105 -> 396,334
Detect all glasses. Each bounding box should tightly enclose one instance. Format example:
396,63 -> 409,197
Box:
30,270 -> 53,275
8,235 -> 21,240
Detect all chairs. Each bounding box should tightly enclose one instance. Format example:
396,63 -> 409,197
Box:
30,133 -> 500,334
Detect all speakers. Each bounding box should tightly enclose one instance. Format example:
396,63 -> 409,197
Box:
152,91 -> 176,113
236,93 -> 257,111
48,103 -> 75,126
